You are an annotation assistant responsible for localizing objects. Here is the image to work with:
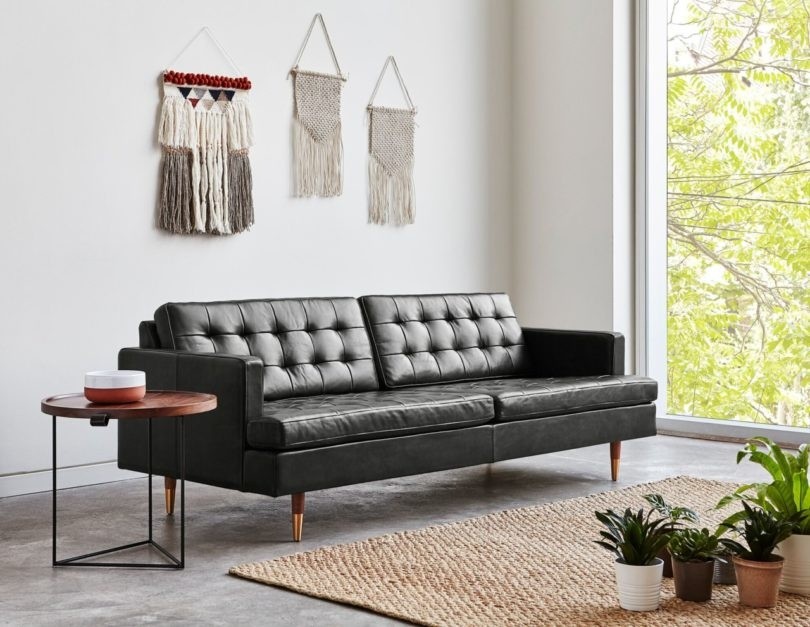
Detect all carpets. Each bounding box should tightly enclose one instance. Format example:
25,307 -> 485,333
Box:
229,476 -> 810,626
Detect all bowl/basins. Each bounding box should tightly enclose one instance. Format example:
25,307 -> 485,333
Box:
84,370 -> 146,403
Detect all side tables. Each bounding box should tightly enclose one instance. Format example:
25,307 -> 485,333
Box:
41,391 -> 217,568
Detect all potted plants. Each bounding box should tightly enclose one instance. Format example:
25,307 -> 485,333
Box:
715,437 -> 810,599
717,500 -> 808,607
640,494 -> 698,577
711,545 -> 739,587
668,525 -> 726,602
593,508 -> 670,611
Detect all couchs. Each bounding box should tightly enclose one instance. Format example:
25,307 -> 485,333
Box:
117,290 -> 657,540
